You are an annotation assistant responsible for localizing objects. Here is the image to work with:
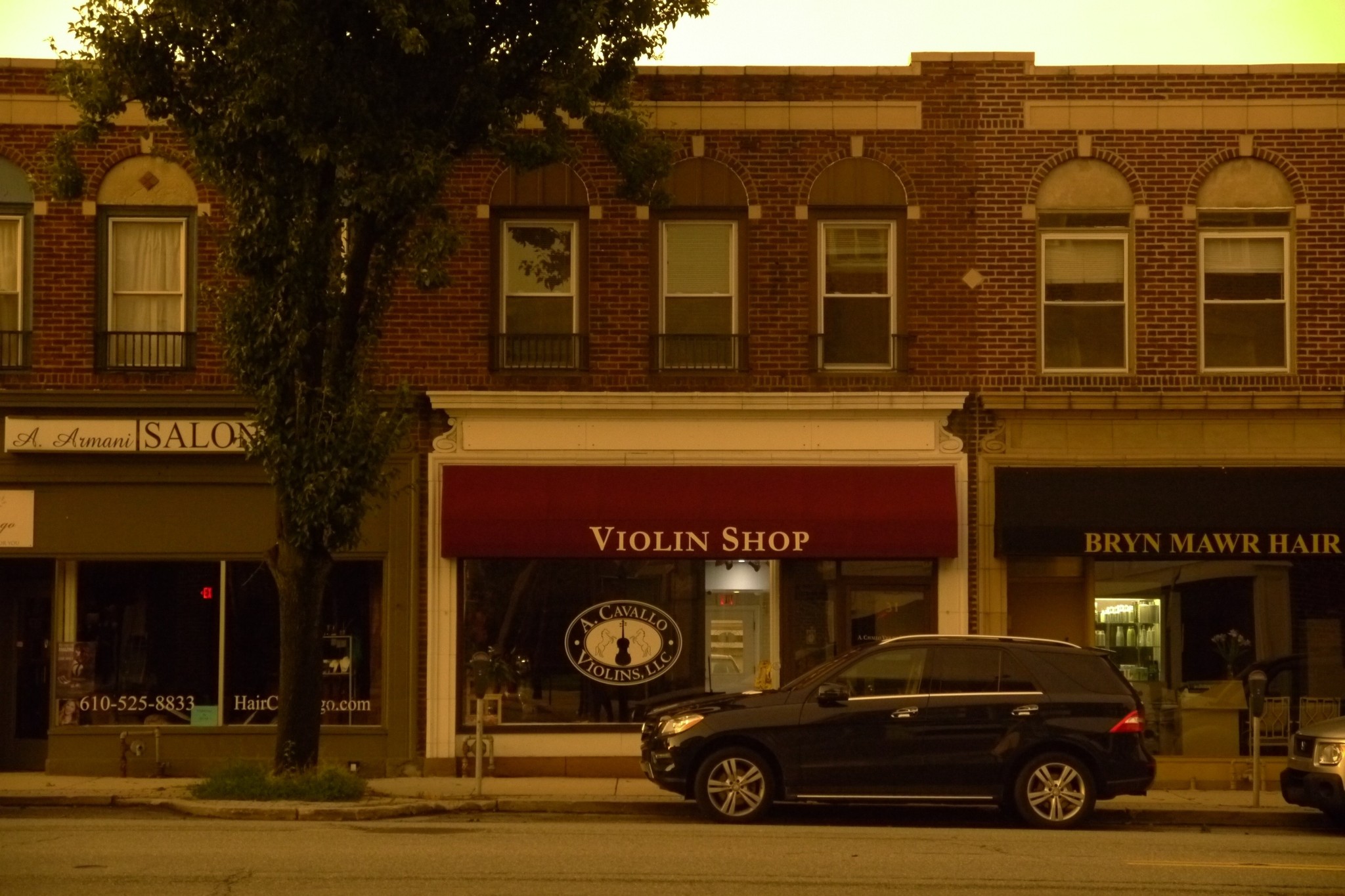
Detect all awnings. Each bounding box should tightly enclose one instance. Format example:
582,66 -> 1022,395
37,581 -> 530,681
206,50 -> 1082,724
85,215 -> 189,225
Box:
440,466 -> 957,560
994,466 -> 1345,560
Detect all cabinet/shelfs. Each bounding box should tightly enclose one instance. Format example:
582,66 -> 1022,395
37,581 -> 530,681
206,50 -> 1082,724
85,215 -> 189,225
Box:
1095,596 -> 1169,711
320,630 -> 358,725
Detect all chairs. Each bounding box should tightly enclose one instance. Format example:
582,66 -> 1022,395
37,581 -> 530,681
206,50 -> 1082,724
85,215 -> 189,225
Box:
464,651 -> 504,723
1294,696 -> 1342,731
1243,693 -> 1290,756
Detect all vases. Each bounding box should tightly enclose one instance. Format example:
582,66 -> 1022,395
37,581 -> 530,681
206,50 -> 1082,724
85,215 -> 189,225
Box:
1223,657 -> 1235,680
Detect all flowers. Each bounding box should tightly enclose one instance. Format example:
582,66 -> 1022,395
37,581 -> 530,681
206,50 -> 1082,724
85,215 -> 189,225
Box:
1210,629 -> 1252,661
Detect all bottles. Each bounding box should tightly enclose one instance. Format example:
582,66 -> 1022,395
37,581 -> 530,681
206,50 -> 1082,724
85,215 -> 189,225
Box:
1127,626 -> 1136,646
1100,604 -> 1134,623
1094,602 -> 1098,623
1136,624 -> 1156,647
1132,601 -> 1159,624
1095,630 -> 1105,646
1116,626 -> 1124,647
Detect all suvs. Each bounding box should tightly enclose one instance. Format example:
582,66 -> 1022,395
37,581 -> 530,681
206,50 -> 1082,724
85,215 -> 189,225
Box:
638,636 -> 1160,831
1280,717 -> 1345,832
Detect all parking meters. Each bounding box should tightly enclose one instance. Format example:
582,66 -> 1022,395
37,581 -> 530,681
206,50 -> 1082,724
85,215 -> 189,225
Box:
1248,670 -> 1267,805
468,652 -> 492,796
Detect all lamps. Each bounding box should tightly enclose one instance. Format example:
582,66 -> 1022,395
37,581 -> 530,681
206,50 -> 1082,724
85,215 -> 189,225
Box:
724,560 -> 733,570
748,560 -> 761,572
